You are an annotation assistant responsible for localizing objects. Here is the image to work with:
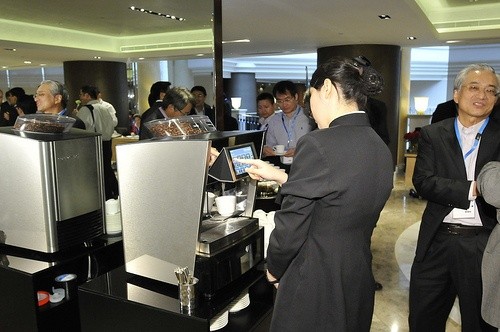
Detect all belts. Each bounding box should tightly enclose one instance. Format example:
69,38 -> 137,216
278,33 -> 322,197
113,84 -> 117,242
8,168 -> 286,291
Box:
441,222 -> 484,236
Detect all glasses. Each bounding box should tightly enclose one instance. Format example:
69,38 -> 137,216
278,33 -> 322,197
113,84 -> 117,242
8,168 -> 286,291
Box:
463,85 -> 497,96
276,97 -> 290,105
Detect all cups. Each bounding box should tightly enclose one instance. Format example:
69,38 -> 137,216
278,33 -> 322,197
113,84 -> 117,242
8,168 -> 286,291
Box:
273,144 -> 285,153
253,209 -> 275,258
177,277 -> 200,315
215,195 -> 235,216
203,191 -> 215,213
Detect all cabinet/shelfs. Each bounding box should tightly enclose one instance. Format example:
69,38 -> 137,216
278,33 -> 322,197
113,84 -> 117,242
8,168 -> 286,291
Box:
0,238 -> 276,332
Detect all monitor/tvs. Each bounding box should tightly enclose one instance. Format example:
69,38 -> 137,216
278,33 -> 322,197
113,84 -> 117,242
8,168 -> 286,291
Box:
210,141 -> 258,182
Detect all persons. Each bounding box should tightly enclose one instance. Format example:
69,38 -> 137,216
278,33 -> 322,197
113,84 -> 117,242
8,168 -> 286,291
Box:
366,95 -> 390,290
140,81 -> 172,128
265,57 -> 394,332
256,93 -> 276,129
0,80 -> 119,200
190,86 -> 215,126
263,81 -> 313,175
139,87 -> 195,139
223,100 -> 238,131
409,64 -> 500,332
410,99 -> 500,201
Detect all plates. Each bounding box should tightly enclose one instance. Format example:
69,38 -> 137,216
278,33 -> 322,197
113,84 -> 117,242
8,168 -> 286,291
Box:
273,151 -> 287,156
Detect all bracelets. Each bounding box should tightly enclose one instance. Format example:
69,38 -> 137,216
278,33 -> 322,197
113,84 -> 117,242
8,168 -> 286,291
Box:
269,279 -> 278,284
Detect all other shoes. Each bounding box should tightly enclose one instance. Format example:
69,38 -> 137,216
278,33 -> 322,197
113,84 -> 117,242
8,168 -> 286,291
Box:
374,280 -> 383,290
